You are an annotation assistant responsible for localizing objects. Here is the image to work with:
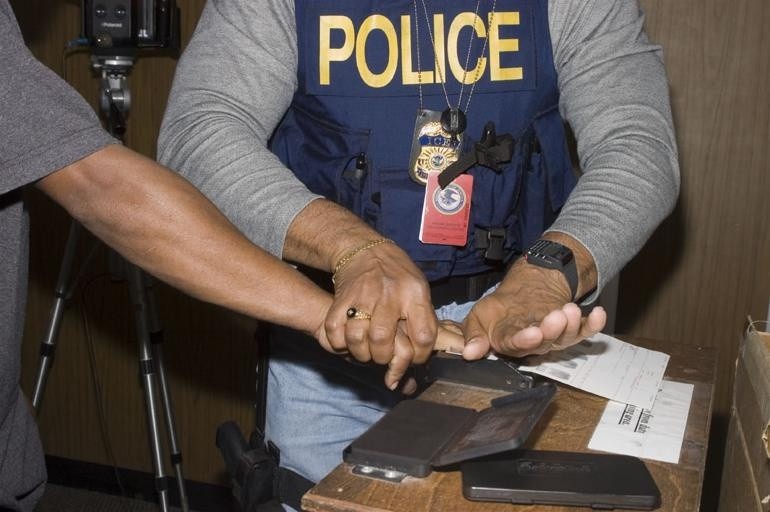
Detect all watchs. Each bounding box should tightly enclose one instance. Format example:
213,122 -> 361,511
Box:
522,240 -> 578,302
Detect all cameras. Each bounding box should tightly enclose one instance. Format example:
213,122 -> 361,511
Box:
63,1 -> 180,58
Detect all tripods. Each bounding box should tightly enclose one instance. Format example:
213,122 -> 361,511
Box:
30,60 -> 191,512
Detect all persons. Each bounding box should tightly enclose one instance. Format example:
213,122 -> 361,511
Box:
1,0 -> 489,512
156,0 -> 680,512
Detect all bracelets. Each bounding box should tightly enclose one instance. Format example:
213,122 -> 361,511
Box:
331,238 -> 396,283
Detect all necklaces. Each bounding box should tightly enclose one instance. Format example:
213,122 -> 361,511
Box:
421,0 -> 478,146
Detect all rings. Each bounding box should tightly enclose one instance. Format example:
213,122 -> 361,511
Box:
344,306 -> 371,320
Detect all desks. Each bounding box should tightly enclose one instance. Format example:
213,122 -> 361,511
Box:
302,336 -> 719,512
